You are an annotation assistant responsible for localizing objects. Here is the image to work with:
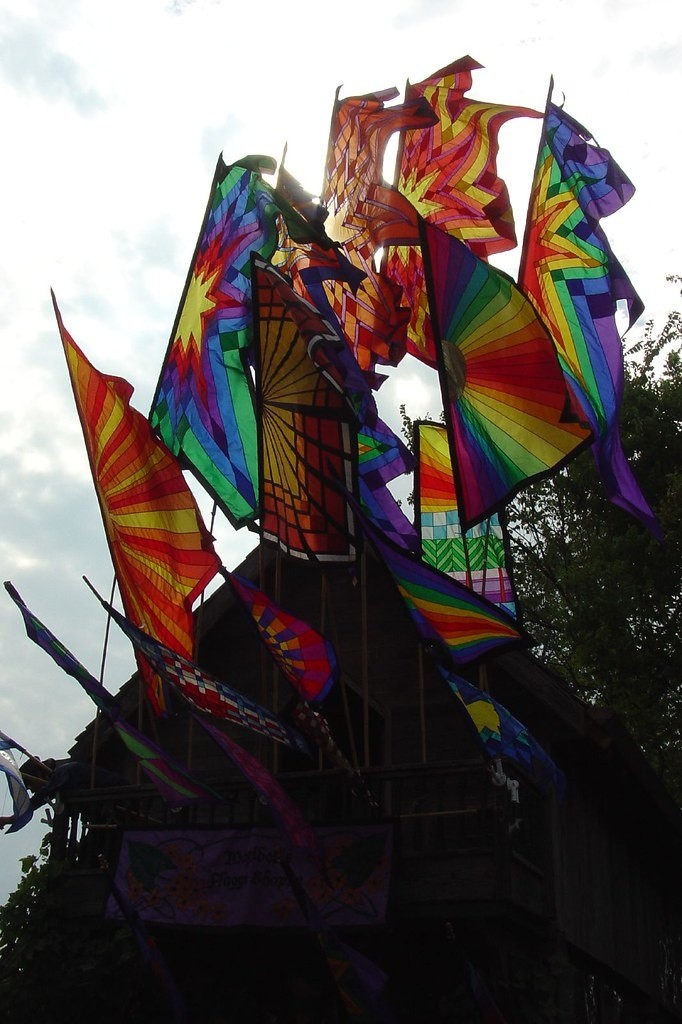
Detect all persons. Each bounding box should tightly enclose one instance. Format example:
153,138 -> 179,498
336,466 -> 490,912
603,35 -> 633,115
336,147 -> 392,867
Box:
0,756 -> 140,843
